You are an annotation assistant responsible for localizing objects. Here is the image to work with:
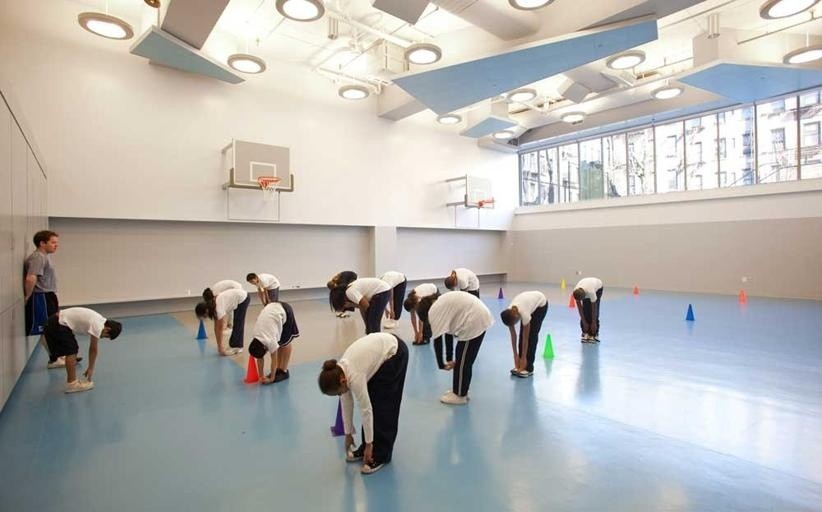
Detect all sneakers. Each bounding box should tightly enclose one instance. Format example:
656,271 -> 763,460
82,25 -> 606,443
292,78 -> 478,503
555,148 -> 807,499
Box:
384,319 -> 400,329
439,392 -> 467,404
59,356 -> 82,362
273,367 -> 289,382
346,443 -> 366,462
225,346 -> 243,355
510,368 -> 518,375
64,379 -> 94,393
587,335 -> 596,343
580,332 -> 588,343
340,310 -> 351,317
335,311 -> 344,317
412,336 -> 429,345
361,456 -> 385,474
518,370 -> 534,378
47,357 -> 66,369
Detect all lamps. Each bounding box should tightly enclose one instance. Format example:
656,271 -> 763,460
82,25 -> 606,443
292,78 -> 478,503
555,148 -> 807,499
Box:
78,0 -> 822,141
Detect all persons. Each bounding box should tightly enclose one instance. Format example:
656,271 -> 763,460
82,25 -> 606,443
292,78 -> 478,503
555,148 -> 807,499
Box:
203,280 -> 243,329
195,289 -> 250,356
318,268 -> 496,474
246,273 -> 280,307
45,307 -> 122,394
573,277 -> 603,343
249,301 -> 300,384
501,290 -> 548,377
22,230 -> 77,368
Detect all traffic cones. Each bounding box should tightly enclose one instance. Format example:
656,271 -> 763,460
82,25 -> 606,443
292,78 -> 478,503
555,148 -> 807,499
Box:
561,279 -> 567,289
196,320 -> 208,338
686,304 -> 695,321
330,397 -> 357,438
543,333 -> 555,359
243,354 -> 259,384
569,295 -> 576,308
633,285 -> 640,295
499,287 -> 504,299
738,289 -> 749,305
